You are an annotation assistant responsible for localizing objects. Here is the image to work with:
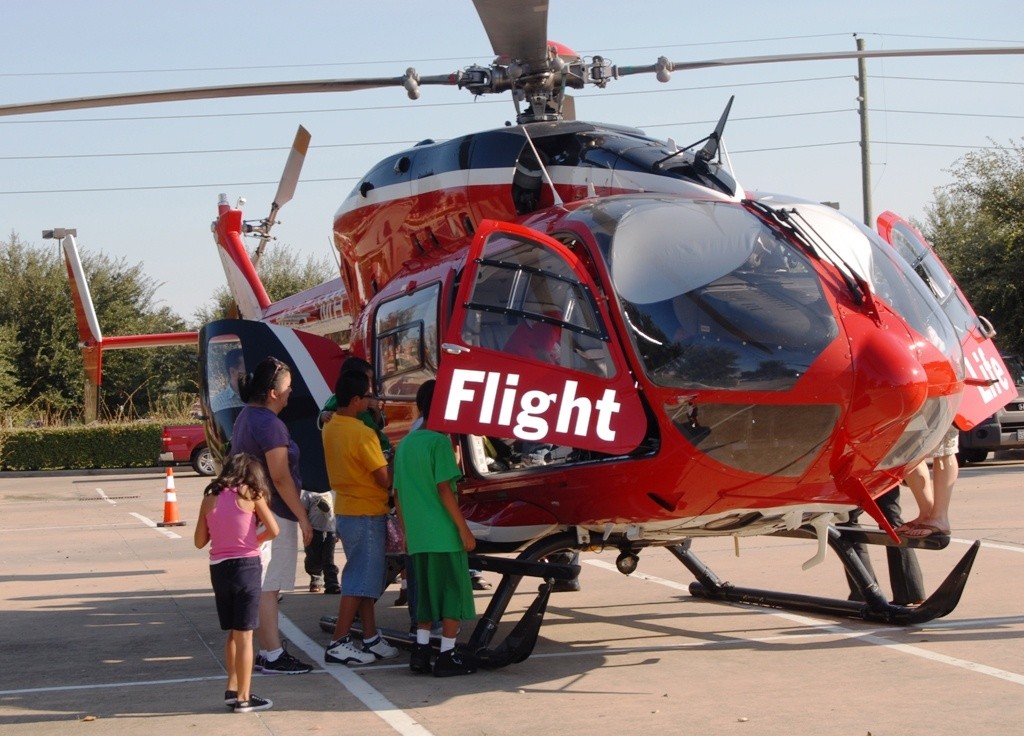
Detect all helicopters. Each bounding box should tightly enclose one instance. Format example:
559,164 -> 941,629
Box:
0,0 -> 1019,666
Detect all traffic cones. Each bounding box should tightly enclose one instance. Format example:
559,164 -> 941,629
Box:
156,468 -> 186,527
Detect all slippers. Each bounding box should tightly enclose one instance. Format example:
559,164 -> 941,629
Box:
893,521 -> 951,539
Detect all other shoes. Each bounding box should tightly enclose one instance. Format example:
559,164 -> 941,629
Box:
538,583 -> 579,592
487,461 -> 508,471
471,575 -> 492,590
521,456 -> 547,469
395,588 -> 408,606
310,586 -> 321,592
325,586 -> 341,594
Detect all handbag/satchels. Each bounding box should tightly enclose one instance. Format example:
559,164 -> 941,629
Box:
385,513 -> 406,551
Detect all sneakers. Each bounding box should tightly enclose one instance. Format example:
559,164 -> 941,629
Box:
325,634 -> 376,665
234,694 -> 273,713
225,690 -> 238,706
361,630 -> 400,659
263,639 -> 313,674
434,649 -> 481,676
409,642 -> 439,672
254,653 -> 265,670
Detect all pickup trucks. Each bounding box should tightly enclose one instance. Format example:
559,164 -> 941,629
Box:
158,423 -> 216,476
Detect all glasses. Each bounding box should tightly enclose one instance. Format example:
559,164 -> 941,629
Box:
358,393 -> 373,399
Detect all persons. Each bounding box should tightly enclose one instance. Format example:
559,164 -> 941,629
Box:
210,349 -> 247,442
194,453 -> 280,713
231,356 -> 314,676
394,566 -> 493,639
321,369 -> 401,666
298,489 -> 342,594
834,485 -> 926,607
317,357 -> 396,515
502,308 -> 563,469
892,424 -> 959,540
392,380 -> 478,678
